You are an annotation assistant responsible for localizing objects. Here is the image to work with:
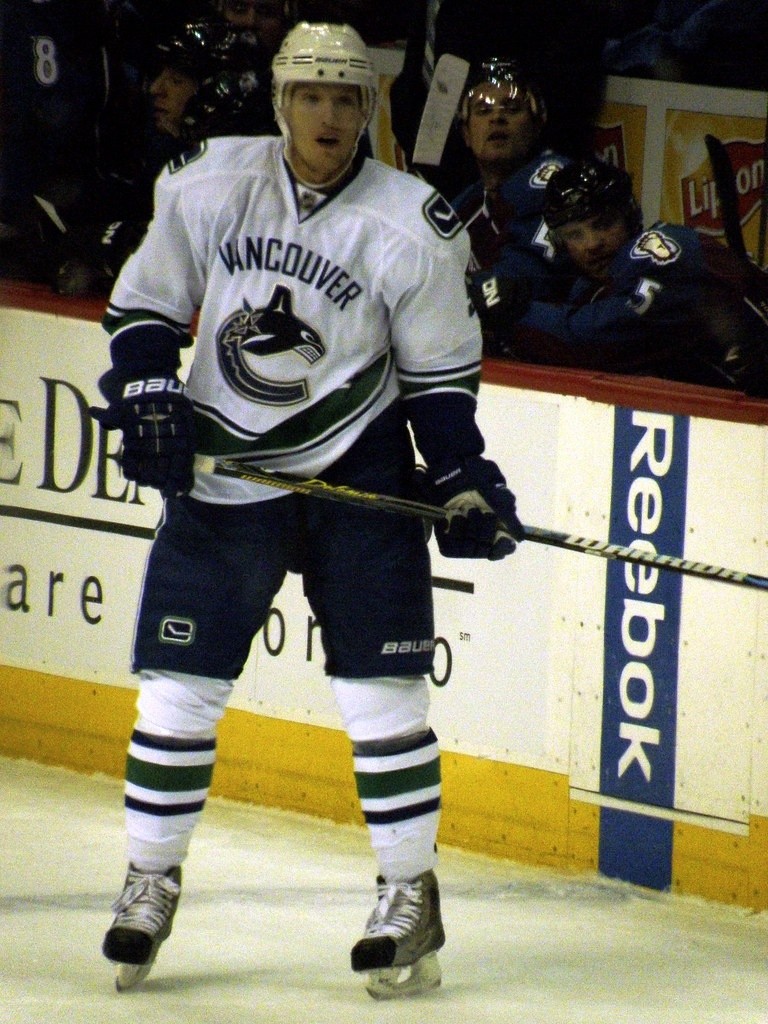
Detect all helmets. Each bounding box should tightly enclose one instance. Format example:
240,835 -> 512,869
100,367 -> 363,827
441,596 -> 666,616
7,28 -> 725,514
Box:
148,16 -> 257,130
540,162 -> 642,256
271,21 -> 377,142
457,54 -> 548,125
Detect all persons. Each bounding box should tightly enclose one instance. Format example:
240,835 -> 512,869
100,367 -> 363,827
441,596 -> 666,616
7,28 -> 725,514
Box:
99,22 -> 526,999
0,0 -> 290,291
392,0 -> 767,395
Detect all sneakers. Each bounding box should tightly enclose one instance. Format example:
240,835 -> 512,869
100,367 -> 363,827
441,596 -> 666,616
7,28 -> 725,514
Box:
352,868 -> 446,1000
101,865 -> 182,993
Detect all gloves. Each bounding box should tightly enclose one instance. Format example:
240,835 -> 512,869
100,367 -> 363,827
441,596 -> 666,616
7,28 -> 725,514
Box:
424,455 -> 525,560
100,368 -> 209,490
472,267 -> 531,327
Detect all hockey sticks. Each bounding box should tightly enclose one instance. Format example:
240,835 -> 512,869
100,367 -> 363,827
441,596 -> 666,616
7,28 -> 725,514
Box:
705,132 -> 762,396
408,52 -> 514,361
193,452 -> 768,591
